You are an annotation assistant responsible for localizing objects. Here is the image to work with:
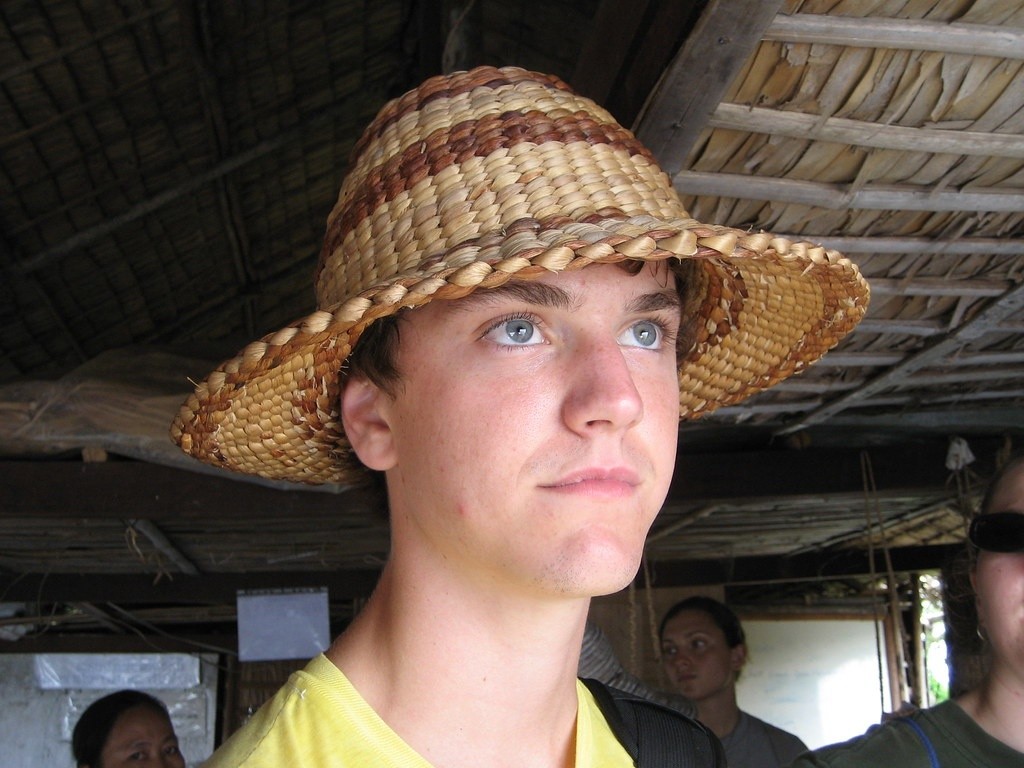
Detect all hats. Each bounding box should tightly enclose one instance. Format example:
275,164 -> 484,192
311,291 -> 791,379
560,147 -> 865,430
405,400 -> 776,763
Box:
170,65 -> 870,488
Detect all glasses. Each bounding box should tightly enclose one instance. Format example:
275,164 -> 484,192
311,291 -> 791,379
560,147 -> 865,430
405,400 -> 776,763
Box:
969,511 -> 1024,554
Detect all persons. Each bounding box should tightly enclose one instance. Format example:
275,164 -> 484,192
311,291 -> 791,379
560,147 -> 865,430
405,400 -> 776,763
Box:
657,595 -> 809,768
169,67 -> 869,768
72,690 -> 185,768
789,450 -> 1024,767
